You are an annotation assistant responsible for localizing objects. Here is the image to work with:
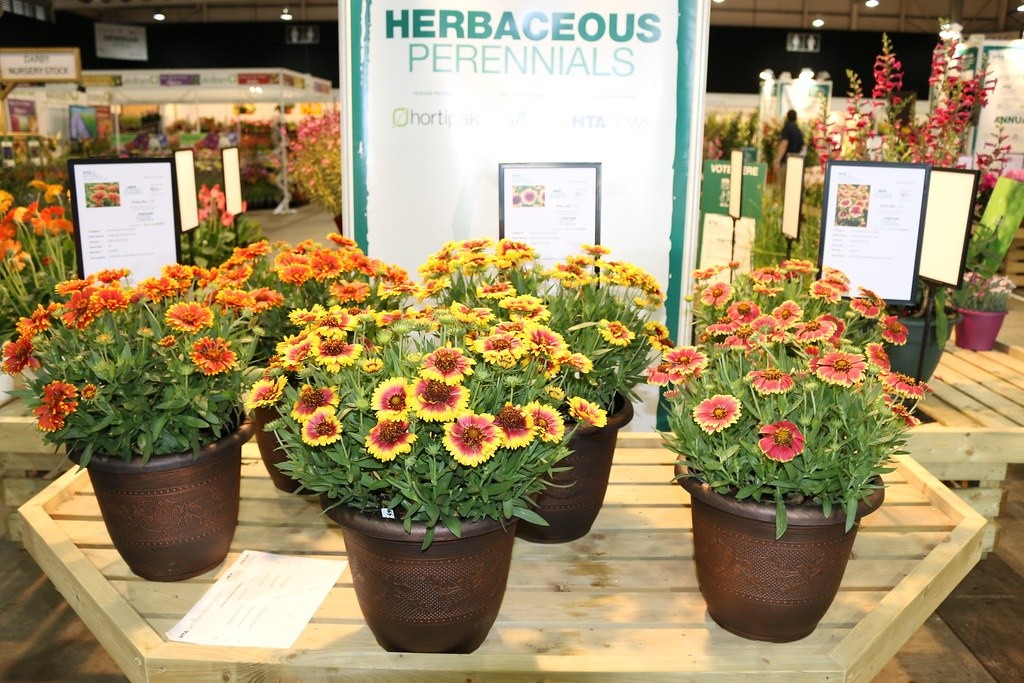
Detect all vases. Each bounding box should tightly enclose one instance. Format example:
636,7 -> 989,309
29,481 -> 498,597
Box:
515,390 -> 633,544
673,452 -> 884,644
254,402 -> 320,495
63,410 -> 252,581
320,493 -> 516,655
955,304 -> 1009,351
879,305 -> 963,387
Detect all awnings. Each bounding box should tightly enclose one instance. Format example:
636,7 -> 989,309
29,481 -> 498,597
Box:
6,68 -> 333,213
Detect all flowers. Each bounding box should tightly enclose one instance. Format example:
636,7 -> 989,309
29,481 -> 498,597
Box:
0,20 -> 1024,551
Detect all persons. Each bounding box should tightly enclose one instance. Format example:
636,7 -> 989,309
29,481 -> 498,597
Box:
124,113 -> 236,154
774,110 -> 804,190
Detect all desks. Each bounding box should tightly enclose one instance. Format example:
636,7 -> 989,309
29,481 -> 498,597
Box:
0,326 -> 1024,552
17,438 -> 989,683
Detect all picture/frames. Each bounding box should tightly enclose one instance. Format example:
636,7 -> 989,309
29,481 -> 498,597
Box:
781,152 -> 806,241
919,166 -> 981,291
728,148 -> 744,219
221,146 -> 243,216
68,159 -> 183,293
816,159 -> 931,308
172,147 -> 201,233
